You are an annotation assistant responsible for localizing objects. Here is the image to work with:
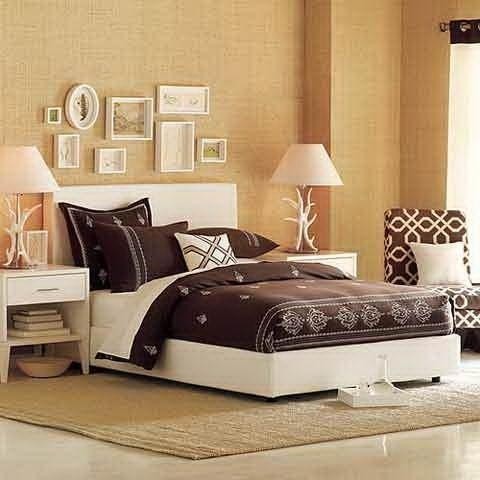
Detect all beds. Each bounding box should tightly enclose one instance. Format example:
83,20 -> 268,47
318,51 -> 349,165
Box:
41,183 -> 463,400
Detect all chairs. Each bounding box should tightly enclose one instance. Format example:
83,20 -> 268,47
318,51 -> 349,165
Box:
382,207 -> 480,352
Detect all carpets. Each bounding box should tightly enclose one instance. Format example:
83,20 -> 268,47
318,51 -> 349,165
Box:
0,350 -> 478,462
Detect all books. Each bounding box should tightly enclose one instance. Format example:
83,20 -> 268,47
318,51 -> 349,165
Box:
7,308 -> 71,338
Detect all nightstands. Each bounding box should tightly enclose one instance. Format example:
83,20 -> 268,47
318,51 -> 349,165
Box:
0,266 -> 94,384
261,249 -> 357,278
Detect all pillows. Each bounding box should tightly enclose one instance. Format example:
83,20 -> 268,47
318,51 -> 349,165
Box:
407,241 -> 470,285
66,201 -> 277,288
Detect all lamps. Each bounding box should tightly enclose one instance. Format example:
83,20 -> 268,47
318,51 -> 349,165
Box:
1,145 -> 56,271
265,142 -> 345,255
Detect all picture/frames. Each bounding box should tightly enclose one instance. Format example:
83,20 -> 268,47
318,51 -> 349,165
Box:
39,84 -> 227,174
